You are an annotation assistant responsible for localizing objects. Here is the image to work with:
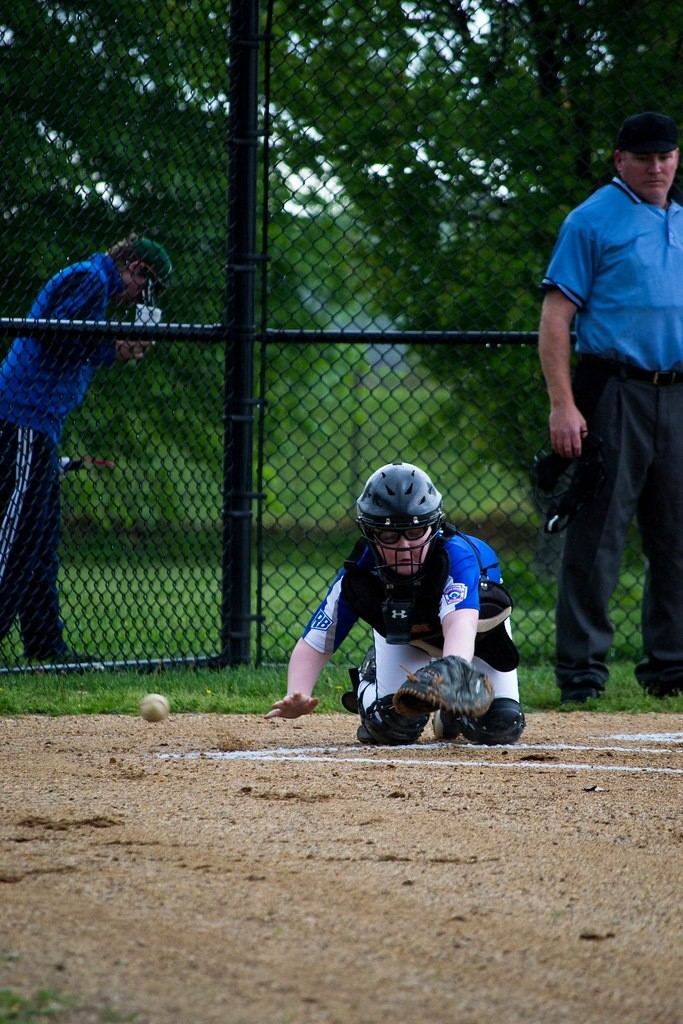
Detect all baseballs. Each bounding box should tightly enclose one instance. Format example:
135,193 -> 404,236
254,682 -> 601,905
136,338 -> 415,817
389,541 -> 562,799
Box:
139,693 -> 171,723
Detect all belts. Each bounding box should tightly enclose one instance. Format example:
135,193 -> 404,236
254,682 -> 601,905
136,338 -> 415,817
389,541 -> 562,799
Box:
581,354 -> 683,386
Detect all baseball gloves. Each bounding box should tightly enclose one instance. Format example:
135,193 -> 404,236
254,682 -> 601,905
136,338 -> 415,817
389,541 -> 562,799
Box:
392,655 -> 495,717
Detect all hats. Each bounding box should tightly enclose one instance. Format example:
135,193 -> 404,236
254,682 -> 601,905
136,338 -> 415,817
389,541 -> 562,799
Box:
617,112 -> 677,153
133,236 -> 172,291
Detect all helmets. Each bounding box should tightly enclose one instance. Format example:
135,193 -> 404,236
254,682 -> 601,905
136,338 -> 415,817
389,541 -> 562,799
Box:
356,462 -> 443,589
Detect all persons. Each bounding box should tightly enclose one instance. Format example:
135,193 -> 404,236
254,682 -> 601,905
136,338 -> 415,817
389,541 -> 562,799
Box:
264,464 -> 527,747
538,111 -> 683,703
0,235 -> 171,665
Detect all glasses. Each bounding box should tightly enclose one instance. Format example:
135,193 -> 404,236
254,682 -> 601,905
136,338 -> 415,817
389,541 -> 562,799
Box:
373,525 -> 429,544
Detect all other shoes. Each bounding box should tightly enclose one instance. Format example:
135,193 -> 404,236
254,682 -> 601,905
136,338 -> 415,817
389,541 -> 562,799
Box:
644,686 -> 683,700
28,653 -> 98,675
433,709 -> 462,742
559,687 -> 599,706
